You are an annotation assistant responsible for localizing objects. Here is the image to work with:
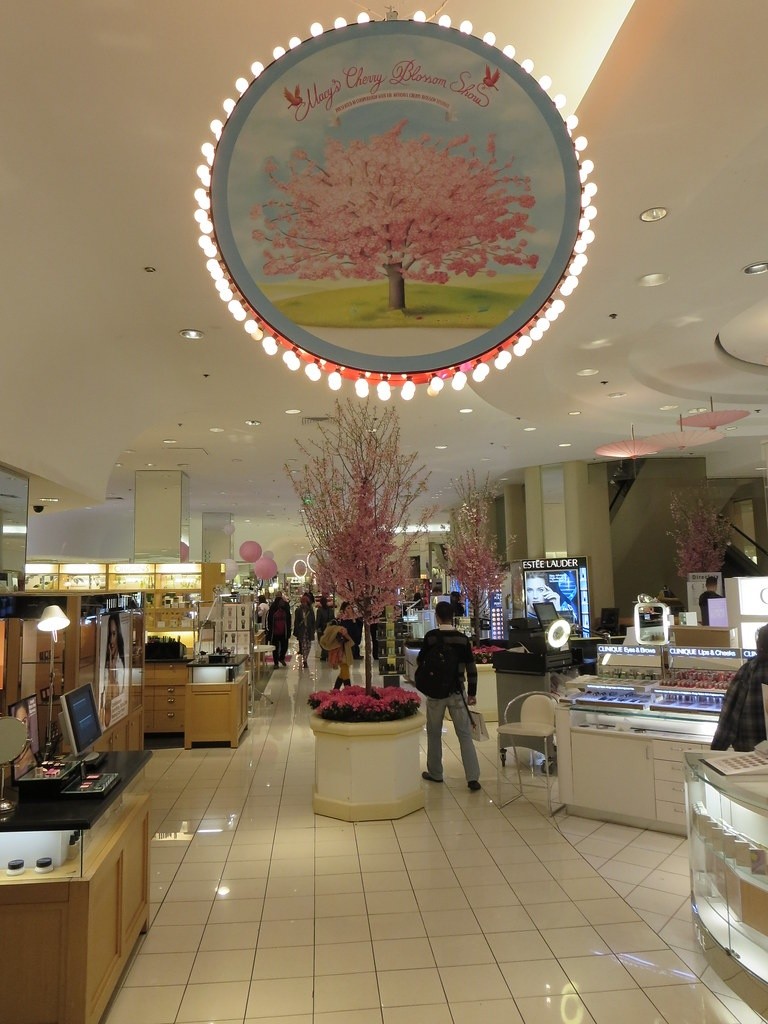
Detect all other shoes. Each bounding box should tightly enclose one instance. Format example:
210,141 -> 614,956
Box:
274,664 -> 279,669
279,657 -> 287,666
467,780 -> 481,791
422,772 -> 443,782
304,662 -> 307,668
354,656 -> 364,659
320,657 -> 326,661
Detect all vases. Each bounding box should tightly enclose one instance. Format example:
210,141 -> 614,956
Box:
308,710 -> 425,822
444,663 -> 499,722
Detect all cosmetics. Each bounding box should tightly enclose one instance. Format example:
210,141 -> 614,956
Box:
42,761 -> 99,788
35,857 -> 53,873
7,860 -> 24,875
598,668 -> 735,704
68,832 -> 80,860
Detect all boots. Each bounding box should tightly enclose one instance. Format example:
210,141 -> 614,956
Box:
334,677 -> 343,690
343,679 -> 352,686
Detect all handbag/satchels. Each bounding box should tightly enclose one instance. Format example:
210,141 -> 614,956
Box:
415,629 -> 458,699
469,703 -> 489,742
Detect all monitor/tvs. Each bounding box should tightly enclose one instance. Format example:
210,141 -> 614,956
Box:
58,681 -> 103,762
533,602 -> 559,630
601,608 -> 619,628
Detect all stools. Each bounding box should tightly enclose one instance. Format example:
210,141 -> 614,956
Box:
496,691 -> 567,817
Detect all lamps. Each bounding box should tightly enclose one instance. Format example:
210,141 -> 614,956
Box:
37,605 -> 71,761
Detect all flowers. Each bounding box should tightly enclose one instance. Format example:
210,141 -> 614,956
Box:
665,482 -> 730,578
438,469 -> 519,665
280,394 -> 434,723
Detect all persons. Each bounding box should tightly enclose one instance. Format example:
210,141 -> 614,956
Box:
316,597 -> 334,661
102,613 -> 125,700
422,602 -> 481,790
710,624 -> 768,753
268,591 -> 291,669
450,592 -> 464,616
294,593 -> 317,668
329,602 -> 363,690
257,595 -> 268,629
526,572 -> 560,618
411,593 -> 424,611
14,701 -> 38,776
699,577 -> 724,626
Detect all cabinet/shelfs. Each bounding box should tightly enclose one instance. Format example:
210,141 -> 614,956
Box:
555,643 -> 758,839
0,563 -> 266,1024
682,750 -> 768,990
376,617 -> 412,676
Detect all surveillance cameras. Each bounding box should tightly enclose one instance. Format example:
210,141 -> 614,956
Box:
33,505 -> 44,513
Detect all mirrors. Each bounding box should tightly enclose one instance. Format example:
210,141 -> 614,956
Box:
634,602 -> 669,646
